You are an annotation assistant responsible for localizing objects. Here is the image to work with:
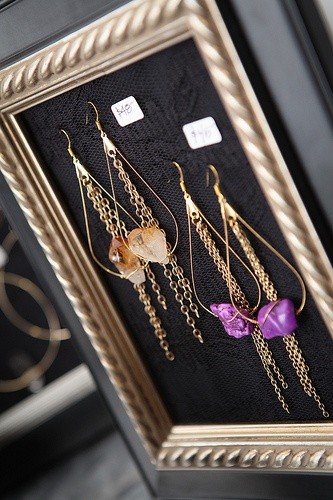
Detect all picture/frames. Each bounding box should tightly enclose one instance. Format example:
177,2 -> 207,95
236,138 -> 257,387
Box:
0,0 -> 333,500
